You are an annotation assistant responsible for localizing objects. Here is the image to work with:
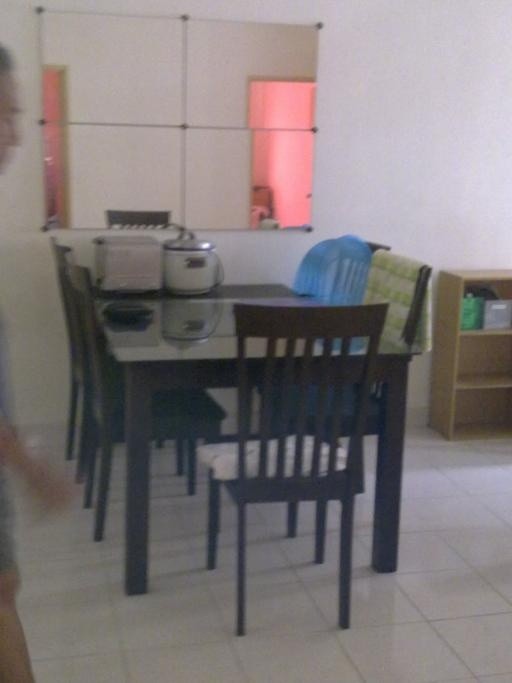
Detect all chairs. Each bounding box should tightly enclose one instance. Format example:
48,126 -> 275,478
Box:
192,301 -> 394,636
341,248 -> 432,363
58,261 -> 232,567
292,235 -> 391,301
51,235 -> 195,489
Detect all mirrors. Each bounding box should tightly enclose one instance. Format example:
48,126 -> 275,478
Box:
37,6 -> 325,231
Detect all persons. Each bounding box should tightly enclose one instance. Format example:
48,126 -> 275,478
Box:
0,45 -> 73,683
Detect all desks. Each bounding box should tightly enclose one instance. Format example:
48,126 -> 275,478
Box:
79,275 -> 426,596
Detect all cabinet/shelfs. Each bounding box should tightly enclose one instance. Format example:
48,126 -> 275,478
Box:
428,270 -> 511,444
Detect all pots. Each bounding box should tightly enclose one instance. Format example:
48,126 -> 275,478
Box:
163,235 -> 218,295
160,296 -> 223,344
